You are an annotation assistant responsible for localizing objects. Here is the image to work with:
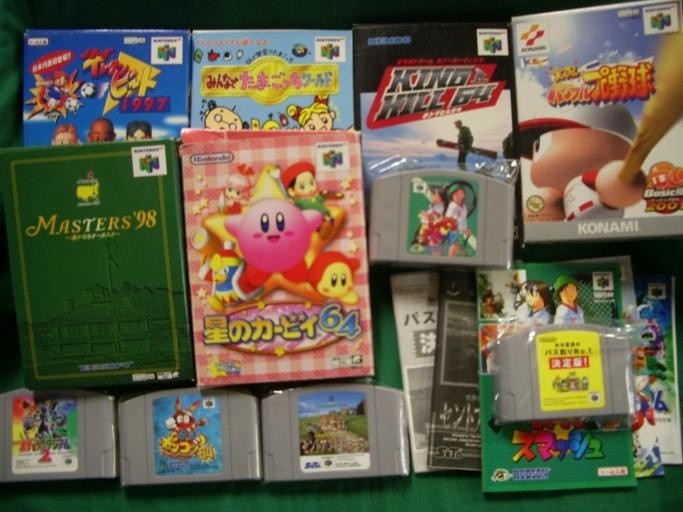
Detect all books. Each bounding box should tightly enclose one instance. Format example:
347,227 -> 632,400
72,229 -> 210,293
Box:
0,0 -> 683,493
